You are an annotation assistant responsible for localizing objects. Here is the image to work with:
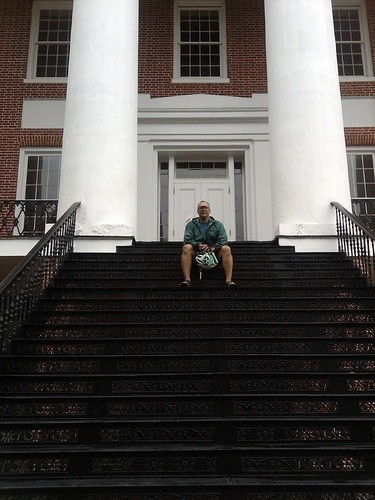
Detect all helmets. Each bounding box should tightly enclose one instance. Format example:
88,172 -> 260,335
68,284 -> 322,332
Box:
196,252 -> 218,269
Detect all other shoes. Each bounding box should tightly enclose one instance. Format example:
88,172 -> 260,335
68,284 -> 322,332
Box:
179,279 -> 193,287
226,280 -> 236,287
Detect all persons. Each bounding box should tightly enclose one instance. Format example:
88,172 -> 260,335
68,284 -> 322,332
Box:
178,200 -> 238,288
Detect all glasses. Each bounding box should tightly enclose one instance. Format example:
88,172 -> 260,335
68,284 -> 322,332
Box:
198,206 -> 209,209
